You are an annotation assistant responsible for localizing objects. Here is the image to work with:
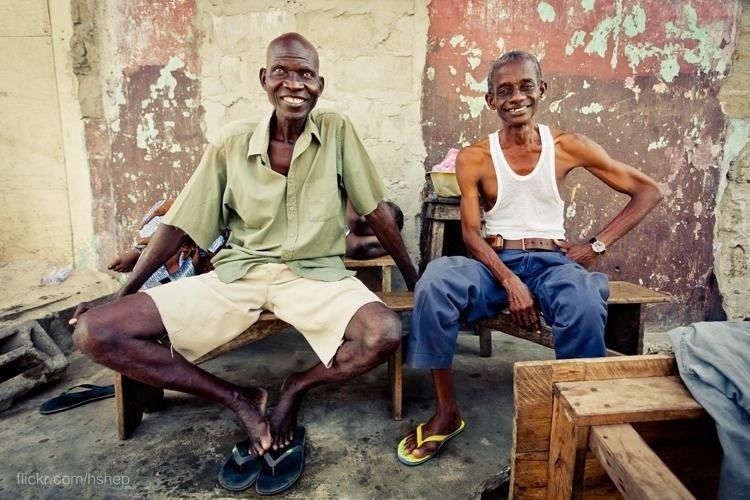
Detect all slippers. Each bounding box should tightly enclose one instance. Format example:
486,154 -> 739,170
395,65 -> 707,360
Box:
397,416 -> 466,466
218,422 -> 308,495
39,383 -> 116,415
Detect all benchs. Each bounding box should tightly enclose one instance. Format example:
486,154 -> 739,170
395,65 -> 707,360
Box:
113,279 -> 670,440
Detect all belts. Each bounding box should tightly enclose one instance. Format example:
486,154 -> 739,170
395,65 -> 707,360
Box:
500,236 -> 570,255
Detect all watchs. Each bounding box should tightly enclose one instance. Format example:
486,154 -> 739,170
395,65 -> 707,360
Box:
588,237 -> 610,257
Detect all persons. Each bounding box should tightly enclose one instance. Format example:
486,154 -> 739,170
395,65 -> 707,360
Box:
105,196 -> 405,289
69,30 -> 420,460
397,48 -> 666,468
